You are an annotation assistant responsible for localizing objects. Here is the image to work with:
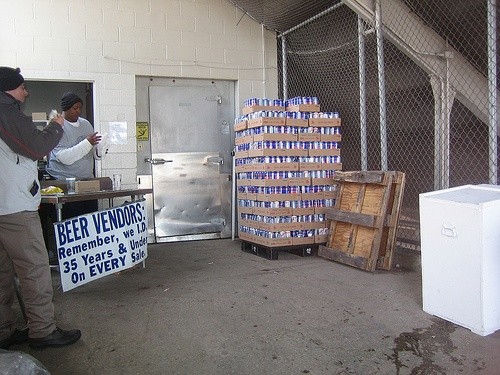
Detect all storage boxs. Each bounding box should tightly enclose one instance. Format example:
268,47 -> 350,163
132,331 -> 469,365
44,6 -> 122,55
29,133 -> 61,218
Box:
419,184 -> 500,336
233,104 -> 342,247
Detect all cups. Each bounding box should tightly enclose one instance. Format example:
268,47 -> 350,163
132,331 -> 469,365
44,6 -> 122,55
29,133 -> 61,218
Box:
66,177 -> 76,195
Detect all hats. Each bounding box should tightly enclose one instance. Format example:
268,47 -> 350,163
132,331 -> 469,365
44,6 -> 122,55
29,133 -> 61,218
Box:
0,67 -> 25,91
61,92 -> 83,112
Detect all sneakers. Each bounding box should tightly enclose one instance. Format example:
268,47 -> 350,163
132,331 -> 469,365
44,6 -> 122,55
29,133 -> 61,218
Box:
28,325 -> 81,349
0,327 -> 30,348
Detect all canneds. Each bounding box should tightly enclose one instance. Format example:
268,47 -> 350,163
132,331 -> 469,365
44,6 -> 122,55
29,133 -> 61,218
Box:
234,96 -> 341,239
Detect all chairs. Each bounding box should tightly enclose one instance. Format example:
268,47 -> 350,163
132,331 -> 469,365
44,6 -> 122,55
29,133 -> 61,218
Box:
75,178 -> 114,209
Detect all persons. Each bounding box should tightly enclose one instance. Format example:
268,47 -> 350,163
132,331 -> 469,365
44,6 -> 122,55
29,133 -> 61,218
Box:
0,66 -> 82,347
44,91 -> 108,220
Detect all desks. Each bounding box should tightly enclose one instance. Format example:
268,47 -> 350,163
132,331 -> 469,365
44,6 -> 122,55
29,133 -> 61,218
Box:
41,189 -> 154,268
